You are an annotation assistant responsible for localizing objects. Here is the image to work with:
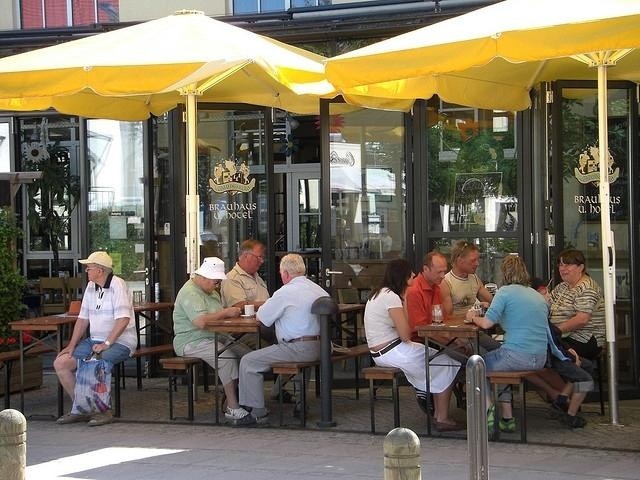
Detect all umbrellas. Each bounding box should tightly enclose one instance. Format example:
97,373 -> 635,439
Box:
327,0 -> 640,429
0,9 -> 339,279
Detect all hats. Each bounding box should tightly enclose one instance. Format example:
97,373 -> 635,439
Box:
78,251 -> 113,268
194,257 -> 228,280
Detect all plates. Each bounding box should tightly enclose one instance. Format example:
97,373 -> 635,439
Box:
241,313 -> 256,318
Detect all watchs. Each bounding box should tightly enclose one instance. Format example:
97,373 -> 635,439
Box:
103,339 -> 113,348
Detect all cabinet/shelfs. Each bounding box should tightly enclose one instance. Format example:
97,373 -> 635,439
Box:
326,251 -> 402,372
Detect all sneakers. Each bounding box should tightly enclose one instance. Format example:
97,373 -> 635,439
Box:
57,411 -> 89,426
498,416 -> 516,432
487,403 -> 496,441
88,410 -> 113,426
542,394 -> 587,429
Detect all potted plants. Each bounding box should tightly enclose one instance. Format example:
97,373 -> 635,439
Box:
0,206 -> 44,394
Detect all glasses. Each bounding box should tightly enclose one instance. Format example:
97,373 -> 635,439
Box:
85,268 -> 93,272
246,252 -> 265,263
461,242 -> 473,252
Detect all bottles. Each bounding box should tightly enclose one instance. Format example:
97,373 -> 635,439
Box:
431,303 -> 444,325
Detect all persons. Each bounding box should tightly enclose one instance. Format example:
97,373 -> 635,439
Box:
171,254 -> 258,421
463,252 -> 551,439
236,251 -> 332,426
529,276 -> 595,427
218,239 -> 298,405
50,248 -> 141,428
440,238 -> 503,358
524,247 -> 608,415
403,247 -> 472,365
361,256 -> 466,433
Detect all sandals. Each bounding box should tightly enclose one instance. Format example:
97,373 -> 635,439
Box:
222,405 -> 272,428
453,382 -> 466,409
292,401 -> 302,417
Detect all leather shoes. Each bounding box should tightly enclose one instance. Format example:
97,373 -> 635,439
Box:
416,396 -> 464,432
270,389 -> 297,402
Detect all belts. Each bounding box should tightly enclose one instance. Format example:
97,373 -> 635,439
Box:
369,338 -> 402,358
288,335 -> 320,343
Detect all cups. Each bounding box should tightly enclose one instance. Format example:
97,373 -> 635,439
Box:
243,304 -> 255,315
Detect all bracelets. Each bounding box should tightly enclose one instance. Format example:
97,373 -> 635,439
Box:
471,314 -> 478,323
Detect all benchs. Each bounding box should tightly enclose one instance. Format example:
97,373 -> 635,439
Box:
487,348 -> 607,442
362,363 -> 405,434
268,343 -> 368,427
161,356 -> 211,421
113,343 -> 174,419
0,344 -> 69,409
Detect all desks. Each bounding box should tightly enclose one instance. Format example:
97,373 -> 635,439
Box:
8,301 -> 173,421
203,304 -> 366,427
274,251 -> 320,286
415,309 -> 503,437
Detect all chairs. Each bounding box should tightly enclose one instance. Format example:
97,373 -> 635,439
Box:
65,279 -> 86,339
37,275 -> 69,339
